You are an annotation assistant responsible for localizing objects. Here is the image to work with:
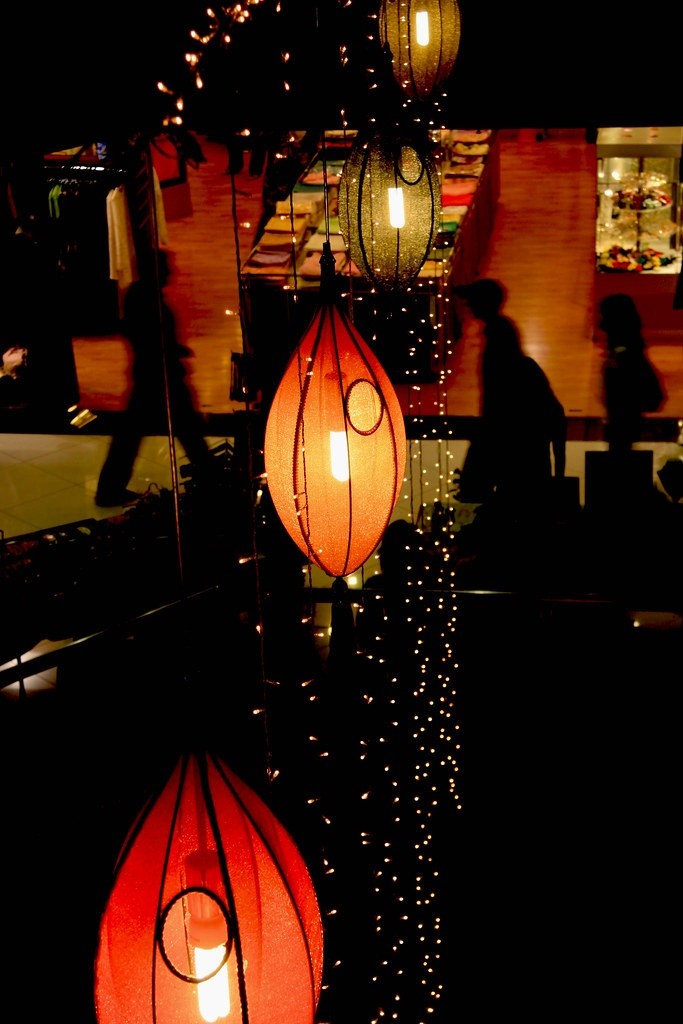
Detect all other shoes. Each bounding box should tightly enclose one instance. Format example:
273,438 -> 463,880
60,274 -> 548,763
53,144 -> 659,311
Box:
95,489 -> 142,507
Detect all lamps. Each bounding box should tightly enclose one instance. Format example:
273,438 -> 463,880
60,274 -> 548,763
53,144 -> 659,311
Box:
341,0 -> 461,297
265,134 -> 409,580
94,148 -> 327,1023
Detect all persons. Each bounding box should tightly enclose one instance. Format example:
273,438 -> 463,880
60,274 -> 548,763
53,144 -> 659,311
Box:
95,246 -> 217,507
463,279 -> 567,504
597,294 -> 665,451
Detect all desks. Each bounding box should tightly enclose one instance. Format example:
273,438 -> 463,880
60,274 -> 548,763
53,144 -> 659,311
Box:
237,128 -> 499,383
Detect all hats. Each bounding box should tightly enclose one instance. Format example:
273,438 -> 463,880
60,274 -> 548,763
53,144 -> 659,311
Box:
456,280 -> 501,301
